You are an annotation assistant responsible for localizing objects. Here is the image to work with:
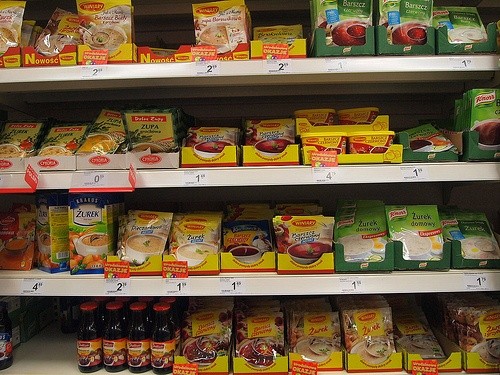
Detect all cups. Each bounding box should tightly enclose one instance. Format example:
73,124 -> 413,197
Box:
58,296 -> 79,333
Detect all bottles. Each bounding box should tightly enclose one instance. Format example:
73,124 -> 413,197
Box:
0,302 -> 14,371
77,296 -> 181,375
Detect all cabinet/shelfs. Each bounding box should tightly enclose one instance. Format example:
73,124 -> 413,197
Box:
1,1 -> 499,373
